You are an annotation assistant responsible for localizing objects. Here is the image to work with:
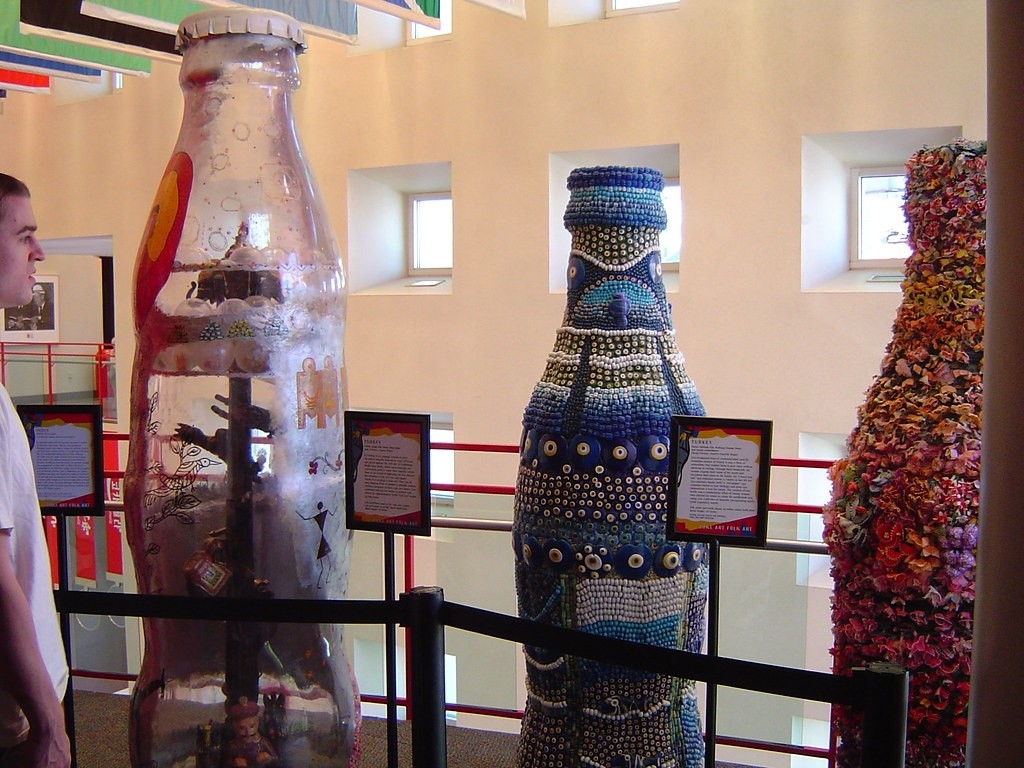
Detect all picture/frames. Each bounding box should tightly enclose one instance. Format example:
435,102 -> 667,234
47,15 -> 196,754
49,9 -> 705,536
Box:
0,274 -> 61,347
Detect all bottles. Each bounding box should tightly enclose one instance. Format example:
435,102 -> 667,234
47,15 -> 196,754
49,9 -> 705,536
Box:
125,8 -> 364,768
515,167 -> 712,768
823,142 -> 988,768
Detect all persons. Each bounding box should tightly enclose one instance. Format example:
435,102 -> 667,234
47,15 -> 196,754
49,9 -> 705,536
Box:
0,174 -> 74,768
